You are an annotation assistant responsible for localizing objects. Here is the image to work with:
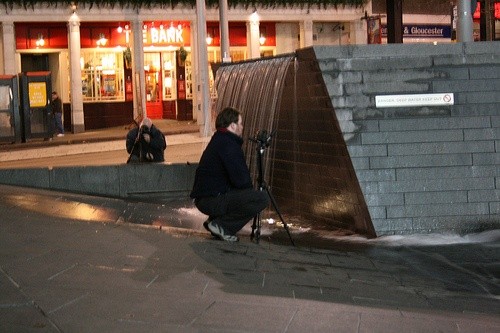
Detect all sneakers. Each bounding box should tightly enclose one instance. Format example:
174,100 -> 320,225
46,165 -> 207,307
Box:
208,220 -> 239,242
57,132 -> 64,136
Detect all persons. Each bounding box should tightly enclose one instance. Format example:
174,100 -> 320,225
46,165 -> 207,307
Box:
126,118 -> 166,164
49,92 -> 65,137
190,107 -> 269,242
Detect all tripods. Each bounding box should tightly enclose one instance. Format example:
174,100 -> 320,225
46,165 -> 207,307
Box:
250,130 -> 296,247
127,129 -> 159,164
